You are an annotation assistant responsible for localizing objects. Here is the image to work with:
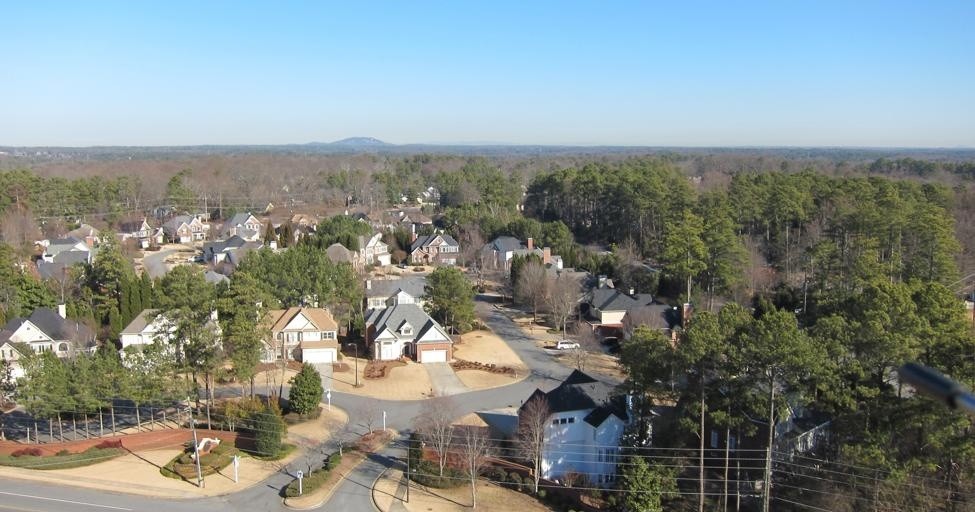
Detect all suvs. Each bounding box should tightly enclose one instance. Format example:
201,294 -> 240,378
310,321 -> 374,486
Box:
555,338 -> 582,351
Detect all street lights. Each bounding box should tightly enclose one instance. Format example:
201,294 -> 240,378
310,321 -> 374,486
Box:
348,342 -> 358,388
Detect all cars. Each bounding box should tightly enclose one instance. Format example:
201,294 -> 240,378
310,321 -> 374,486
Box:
399,263 -> 408,269
188,255 -> 202,262
413,265 -> 426,272
